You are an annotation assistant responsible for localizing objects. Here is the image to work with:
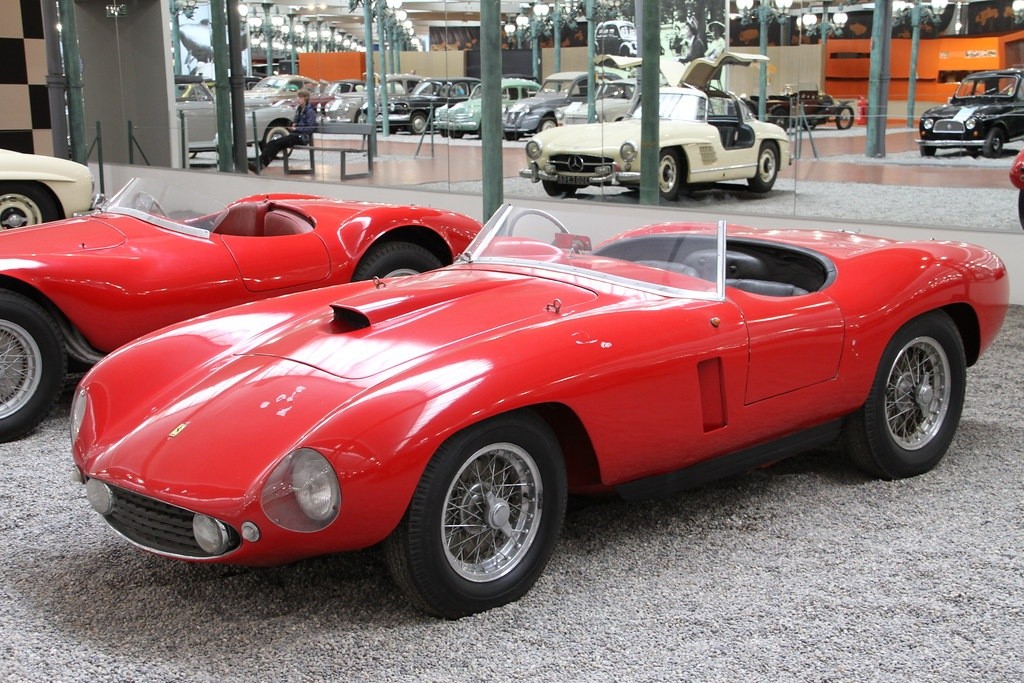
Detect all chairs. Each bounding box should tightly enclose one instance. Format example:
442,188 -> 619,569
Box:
263,210 -> 313,236
683,249 -> 774,282
725,279 -> 809,297
214,201 -> 269,236
634,260 -> 700,278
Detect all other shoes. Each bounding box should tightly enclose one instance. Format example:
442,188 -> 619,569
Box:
248,162 -> 262,175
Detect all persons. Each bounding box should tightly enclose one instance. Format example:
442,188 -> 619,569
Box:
678,16 -> 706,63
248,90 -> 316,176
669,24 -> 689,56
704,21 -> 726,62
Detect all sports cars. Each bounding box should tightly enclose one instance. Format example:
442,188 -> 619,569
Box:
69,204 -> 1010,620
0,176 -> 486,444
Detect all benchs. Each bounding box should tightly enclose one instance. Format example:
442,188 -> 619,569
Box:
283,123 -> 374,181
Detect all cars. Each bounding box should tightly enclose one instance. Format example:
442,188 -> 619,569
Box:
914,68 -> 1024,158
308,79 -> 365,113
245,75 -> 318,105
324,73 -> 423,123
503,71 -> 634,141
359,77 -> 482,136
518,49 -> 789,202
594,20 -> 665,59
435,78 -> 542,141
0,143 -> 95,231
174,74 -> 297,161
